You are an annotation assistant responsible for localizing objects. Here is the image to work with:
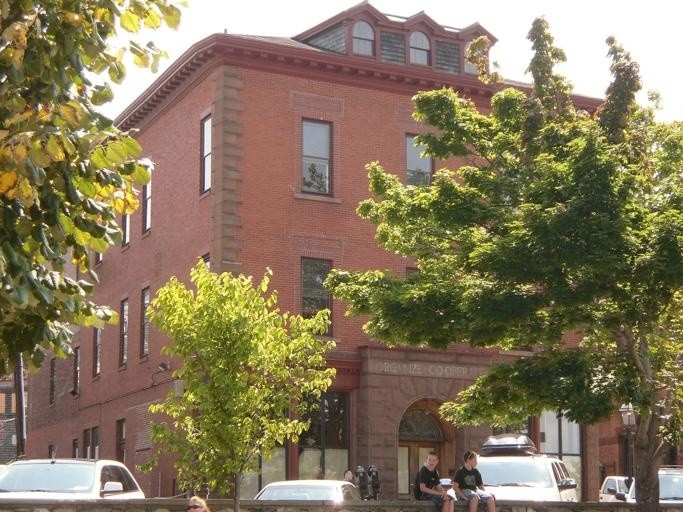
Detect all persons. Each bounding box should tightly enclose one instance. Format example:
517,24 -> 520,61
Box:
342,469 -> 353,482
599,460 -> 606,489
412,450 -> 454,511
451,449 -> 495,512
186,495 -> 210,512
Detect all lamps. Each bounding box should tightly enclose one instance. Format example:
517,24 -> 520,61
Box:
158,362 -> 168,371
69,388 -> 80,399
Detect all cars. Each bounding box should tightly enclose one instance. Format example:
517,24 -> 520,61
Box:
251,479 -> 361,502
0,459 -> 145,501
600,467 -> 683,503
441,433 -> 579,503
